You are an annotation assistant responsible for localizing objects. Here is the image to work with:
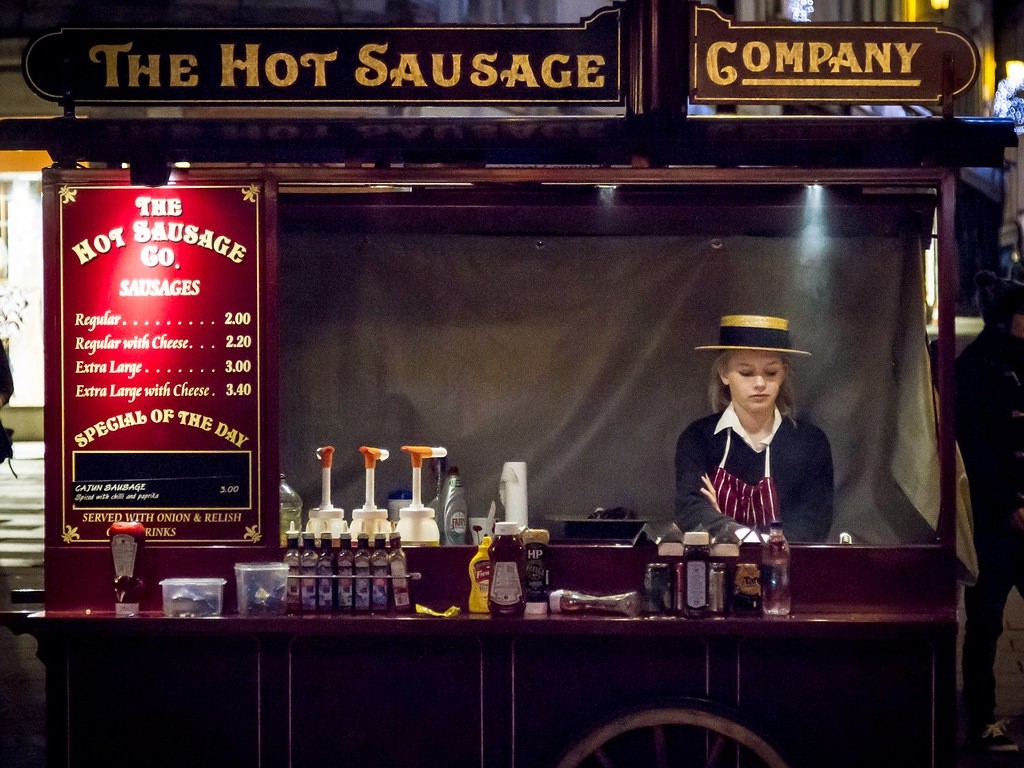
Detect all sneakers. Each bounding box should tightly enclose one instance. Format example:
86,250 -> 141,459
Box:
964,718 -> 1018,752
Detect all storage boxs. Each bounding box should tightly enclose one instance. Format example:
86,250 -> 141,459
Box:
234,561 -> 291,614
159,578 -> 228,618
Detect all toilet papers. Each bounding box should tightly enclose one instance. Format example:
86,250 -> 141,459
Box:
500,461 -> 529,530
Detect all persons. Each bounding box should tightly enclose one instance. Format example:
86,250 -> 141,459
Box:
675,315 -> 833,546
951,270 -> 1024,753
0,340 -> 14,409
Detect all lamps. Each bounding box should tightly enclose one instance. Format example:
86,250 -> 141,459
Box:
129,156 -> 172,187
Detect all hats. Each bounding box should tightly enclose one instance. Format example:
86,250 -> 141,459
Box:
695,314 -> 813,357
971,270 -> 1023,325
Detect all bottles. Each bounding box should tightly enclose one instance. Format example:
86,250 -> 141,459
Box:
469,520 -> 549,616
109,521 -> 146,616
279,474 -> 303,545
438,465 -> 468,545
681,520 -> 791,615
551,589 -> 643,616
284,522 -> 412,615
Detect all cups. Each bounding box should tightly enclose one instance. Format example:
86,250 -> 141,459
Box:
468,517 -> 500,545
387,491 -> 412,522
